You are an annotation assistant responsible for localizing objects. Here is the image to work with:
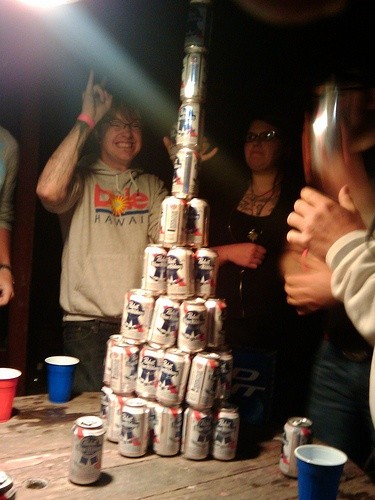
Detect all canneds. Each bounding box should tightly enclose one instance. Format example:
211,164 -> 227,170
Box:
102,196 -> 240,461
180,53 -> 208,104
184,1 -> 211,54
69,415 -> 107,485
171,148 -> 201,200
0,470 -> 15,500
278,418 -> 312,478
175,99 -> 204,150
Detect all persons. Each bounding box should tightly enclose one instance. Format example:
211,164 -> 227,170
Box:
279,39 -> 375,485
0,123 -> 20,358
36,69 -> 217,394
206,109 -> 304,426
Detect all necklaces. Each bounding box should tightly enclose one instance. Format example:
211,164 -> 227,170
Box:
241,180 -> 280,242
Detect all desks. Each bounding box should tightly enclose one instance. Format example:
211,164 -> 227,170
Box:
0,391 -> 375,500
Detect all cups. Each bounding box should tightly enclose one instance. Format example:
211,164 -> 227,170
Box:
293,443 -> 347,500
44,356 -> 80,403
0,367 -> 21,423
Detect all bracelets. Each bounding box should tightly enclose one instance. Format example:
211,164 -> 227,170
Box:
0,265 -> 12,270
77,113 -> 97,130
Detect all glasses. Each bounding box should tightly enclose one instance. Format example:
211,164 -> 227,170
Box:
106,118 -> 145,132
244,129 -> 281,142
